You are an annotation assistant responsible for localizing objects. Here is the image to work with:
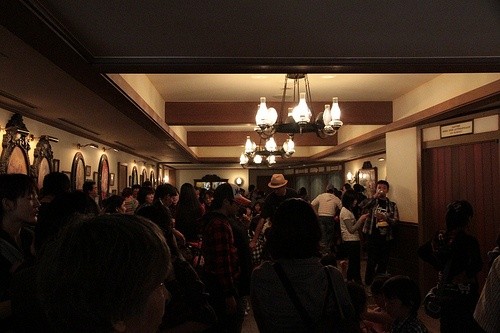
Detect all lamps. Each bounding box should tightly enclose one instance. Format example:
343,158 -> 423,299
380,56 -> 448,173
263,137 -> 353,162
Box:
31,134 -> 59,142
78,143 -> 98,149
346,171 -> 356,184
255,70 -> 343,139
238,135 -> 296,170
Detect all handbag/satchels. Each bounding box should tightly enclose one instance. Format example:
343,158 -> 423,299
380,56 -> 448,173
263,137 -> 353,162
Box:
423,287 -> 440,319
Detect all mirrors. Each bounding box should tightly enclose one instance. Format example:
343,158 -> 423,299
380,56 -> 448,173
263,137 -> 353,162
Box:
357,168 -> 377,195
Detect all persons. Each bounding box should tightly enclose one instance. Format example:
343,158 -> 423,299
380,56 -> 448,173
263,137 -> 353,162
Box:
418,199 -> 482,333
36,214 -> 171,333
250,198 -> 356,333
1,173 -> 500,333
383,276 -> 428,333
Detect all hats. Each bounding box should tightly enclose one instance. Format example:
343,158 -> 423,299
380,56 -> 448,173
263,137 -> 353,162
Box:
213,183 -> 252,206
268,174 -> 288,188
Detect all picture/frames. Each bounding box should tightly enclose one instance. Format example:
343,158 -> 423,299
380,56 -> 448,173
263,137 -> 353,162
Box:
0,113 -> 154,209
